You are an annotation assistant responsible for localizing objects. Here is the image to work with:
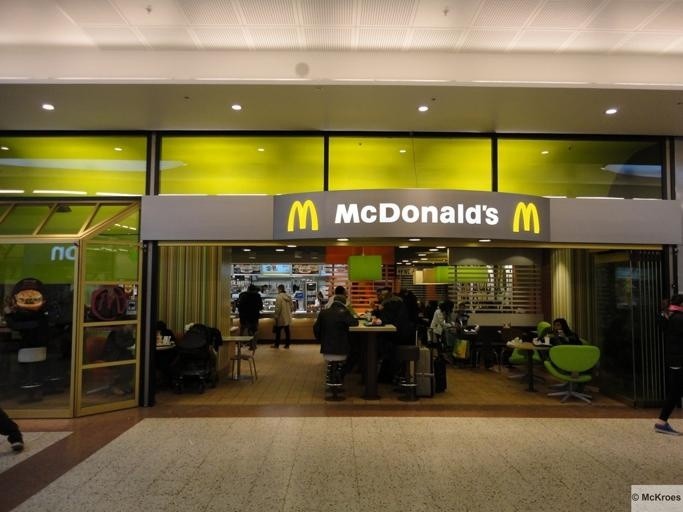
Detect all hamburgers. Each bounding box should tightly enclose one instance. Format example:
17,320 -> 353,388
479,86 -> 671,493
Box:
17,290 -> 43,309
234,264 -> 259,274
292,264 -> 318,274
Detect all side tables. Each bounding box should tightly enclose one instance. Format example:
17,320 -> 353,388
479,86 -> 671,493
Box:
215,336 -> 255,380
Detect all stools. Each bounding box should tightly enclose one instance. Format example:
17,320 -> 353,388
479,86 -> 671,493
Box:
324,354 -> 349,401
397,345 -> 420,401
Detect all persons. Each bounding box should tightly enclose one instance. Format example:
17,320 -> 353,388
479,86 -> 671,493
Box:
0,408 -> 24,451
323,285 -> 349,310
314,291 -> 324,306
100,324 -> 136,395
312,294 -> 361,367
235,284 -> 263,349
653,290 -> 683,435
270,284 -> 295,348
553,318 -> 583,346
370,286 -> 516,347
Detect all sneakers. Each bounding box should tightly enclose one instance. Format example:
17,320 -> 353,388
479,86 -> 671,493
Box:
8,431 -> 24,450
240,341 -> 289,350
325,389 -> 346,401
655,422 -> 680,436
110,382 -> 131,395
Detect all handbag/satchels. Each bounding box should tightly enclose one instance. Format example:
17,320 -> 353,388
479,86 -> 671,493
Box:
272,324 -> 280,333
452,338 -> 470,359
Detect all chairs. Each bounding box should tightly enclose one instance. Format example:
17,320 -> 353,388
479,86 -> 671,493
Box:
230,331 -> 258,384
543,338 -> 600,405
509,321 -> 552,384
86,336 -> 113,395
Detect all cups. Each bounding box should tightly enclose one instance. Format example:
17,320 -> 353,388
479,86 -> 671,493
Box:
545,337 -> 550,344
